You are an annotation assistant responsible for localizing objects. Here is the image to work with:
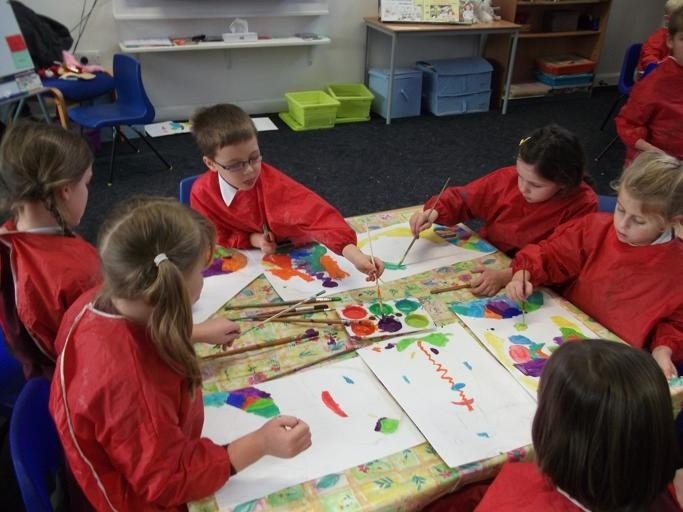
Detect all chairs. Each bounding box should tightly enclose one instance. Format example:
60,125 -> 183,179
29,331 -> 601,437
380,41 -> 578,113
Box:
41,64 -> 115,136
8,377 -> 95,512
70,53 -> 172,187
178,175 -> 201,207
0,328 -> 28,418
595,195 -> 620,213
593,63 -> 658,162
599,43 -> 643,132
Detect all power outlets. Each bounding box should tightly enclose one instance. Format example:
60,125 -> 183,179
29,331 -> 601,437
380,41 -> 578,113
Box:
70,49 -> 101,68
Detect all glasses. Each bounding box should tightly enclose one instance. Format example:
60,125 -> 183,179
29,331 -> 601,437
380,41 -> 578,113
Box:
212,151 -> 261,171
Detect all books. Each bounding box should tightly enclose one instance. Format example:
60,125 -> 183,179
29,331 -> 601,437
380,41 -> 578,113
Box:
123,38 -> 172,48
143,119 -> 192,138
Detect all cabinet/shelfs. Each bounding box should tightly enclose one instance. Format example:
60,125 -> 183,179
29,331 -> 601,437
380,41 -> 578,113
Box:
491,1 -> 614,115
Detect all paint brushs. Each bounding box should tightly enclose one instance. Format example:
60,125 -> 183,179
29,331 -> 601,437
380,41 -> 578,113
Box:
251,317 -> 367,327
230,304 -> 328,321
398,177 -> 451,267
522,260 -> 526,324
366,223 -> 386,323
199,330 -> 319,361
255,308 -> 336,318
238,290 -> 325,338
225,297 -> 342,311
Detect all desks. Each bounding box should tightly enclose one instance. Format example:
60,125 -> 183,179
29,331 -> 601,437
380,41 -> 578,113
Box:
363,14 -> 524,124
187,206 -> 683,511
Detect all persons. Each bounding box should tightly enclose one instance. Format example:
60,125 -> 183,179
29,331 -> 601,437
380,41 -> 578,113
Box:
409,123 -> 599,298
48,194 -> 313,511
637,0 -> 683,78
189,103 -> 385,282
616,5 -> 682,172
504,150 -> 682,378
462,4 -> 474,24
477,339 -> 682,512
0,117 -> 103,381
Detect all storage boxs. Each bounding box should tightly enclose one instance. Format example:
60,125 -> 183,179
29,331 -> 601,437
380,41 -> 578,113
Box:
325,83 -> 376,122
370,65 -> 423,119
417,55 -> 495,117
278,90 -> 340,130
